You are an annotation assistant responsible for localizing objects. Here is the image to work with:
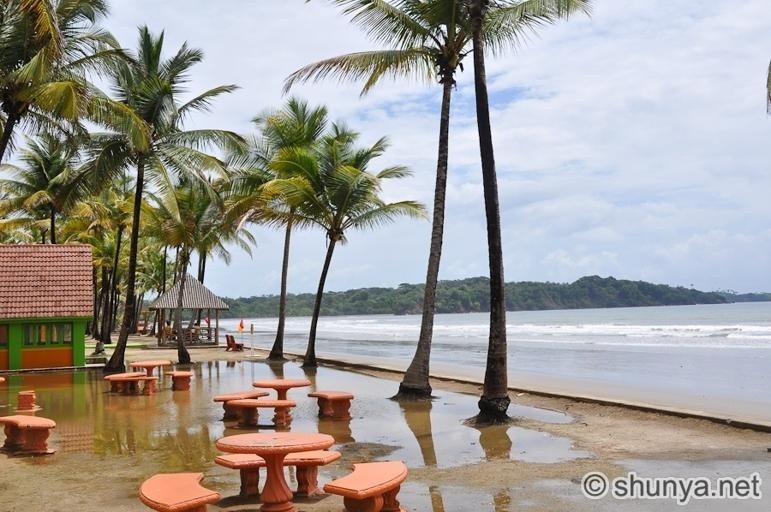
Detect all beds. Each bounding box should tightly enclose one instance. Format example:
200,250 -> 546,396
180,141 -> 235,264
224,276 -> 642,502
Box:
128,359 -> 171,395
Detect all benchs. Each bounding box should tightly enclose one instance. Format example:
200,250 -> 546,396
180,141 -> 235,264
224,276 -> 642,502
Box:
1,371 -> 408,512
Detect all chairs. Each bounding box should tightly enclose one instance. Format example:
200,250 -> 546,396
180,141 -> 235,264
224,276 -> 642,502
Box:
225,335 -> 244,352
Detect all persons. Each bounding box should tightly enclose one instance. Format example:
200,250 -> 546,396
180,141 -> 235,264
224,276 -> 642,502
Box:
191,323 -> 203,342
164,324 -> 172,339
